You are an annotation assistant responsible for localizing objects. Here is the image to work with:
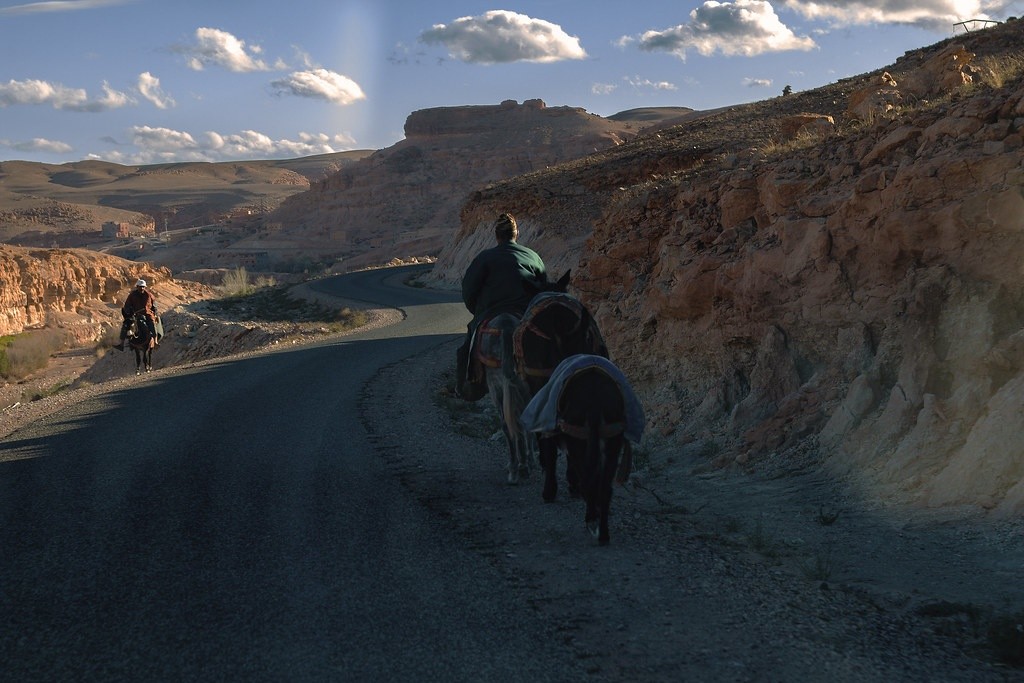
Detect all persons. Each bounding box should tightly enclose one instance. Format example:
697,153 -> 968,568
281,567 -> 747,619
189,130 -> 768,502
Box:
457,214 -> 549,401
112,279 -> 161,351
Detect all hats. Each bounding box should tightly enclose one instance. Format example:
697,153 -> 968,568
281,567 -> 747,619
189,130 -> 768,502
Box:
136,279 -> 148,287
497,212 -> 517,230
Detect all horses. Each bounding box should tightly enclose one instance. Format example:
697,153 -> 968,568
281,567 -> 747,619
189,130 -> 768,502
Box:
473,267 -> 628,550
120,307 -> 156,376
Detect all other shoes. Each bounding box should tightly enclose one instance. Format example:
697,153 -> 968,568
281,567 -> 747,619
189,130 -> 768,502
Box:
112,344 -> 125,352
155,342 -> 161,348
